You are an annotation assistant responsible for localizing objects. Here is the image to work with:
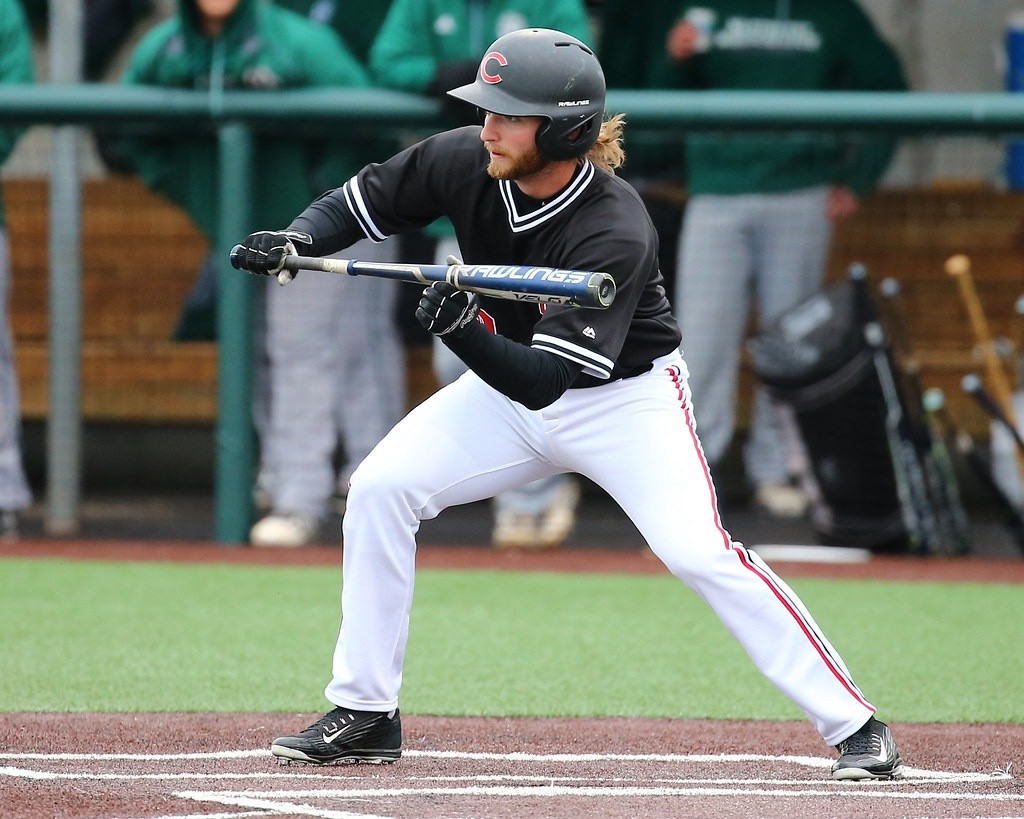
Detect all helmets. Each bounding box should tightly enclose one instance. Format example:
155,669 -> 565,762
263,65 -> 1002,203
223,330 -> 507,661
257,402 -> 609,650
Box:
446,27 -> 606,160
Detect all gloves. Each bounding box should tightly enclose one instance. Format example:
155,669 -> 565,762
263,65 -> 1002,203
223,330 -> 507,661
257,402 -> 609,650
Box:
237,229 -> 313,286
415,255 -> 481,345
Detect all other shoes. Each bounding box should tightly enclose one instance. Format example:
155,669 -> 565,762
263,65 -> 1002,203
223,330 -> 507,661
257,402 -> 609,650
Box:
494,509 -> 536,546
758,485 -> 812,520
538,481 -> 581,545
249,510 -> 321,547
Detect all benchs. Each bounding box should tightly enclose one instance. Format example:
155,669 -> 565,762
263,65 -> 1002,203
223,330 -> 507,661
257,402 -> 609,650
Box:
0,171 -> 1024,435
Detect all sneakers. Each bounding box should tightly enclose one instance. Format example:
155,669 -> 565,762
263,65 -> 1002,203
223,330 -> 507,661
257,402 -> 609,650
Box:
271,705 -> 403,763
831,718 -> 903,779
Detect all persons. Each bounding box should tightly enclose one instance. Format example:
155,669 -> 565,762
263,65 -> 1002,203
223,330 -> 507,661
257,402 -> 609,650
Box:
231,27 -> 902,780
0,1 -> 906,550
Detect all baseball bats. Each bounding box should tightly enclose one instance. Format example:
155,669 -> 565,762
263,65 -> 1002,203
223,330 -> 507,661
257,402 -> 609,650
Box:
846,252 -> 1024,556
229,244 -> 617,311
